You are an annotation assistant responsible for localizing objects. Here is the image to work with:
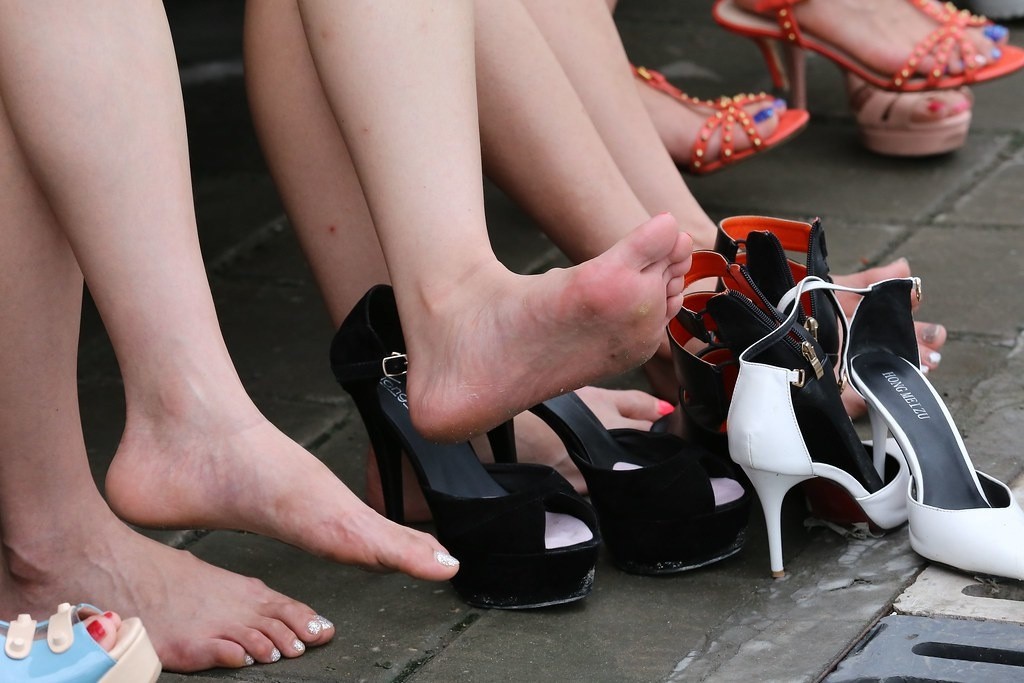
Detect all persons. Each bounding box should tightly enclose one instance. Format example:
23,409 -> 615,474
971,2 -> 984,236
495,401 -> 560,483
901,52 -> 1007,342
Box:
241,0 -> 1024,525
1,0 -> 461,674
0,601 -> 162,683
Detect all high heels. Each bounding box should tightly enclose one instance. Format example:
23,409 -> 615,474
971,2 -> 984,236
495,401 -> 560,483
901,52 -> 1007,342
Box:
727,274 -> 913,579
486,388 -> 753,577
712,0 -> 1024,91
842,276 -> 1024,582
786,44 -> 974,155
327,283 -> 603,610
629,58 -> 810,175
663,214 -> 885,496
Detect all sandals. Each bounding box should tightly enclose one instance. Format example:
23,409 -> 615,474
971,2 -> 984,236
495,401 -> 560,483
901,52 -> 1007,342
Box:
0,602 -> 163,683
